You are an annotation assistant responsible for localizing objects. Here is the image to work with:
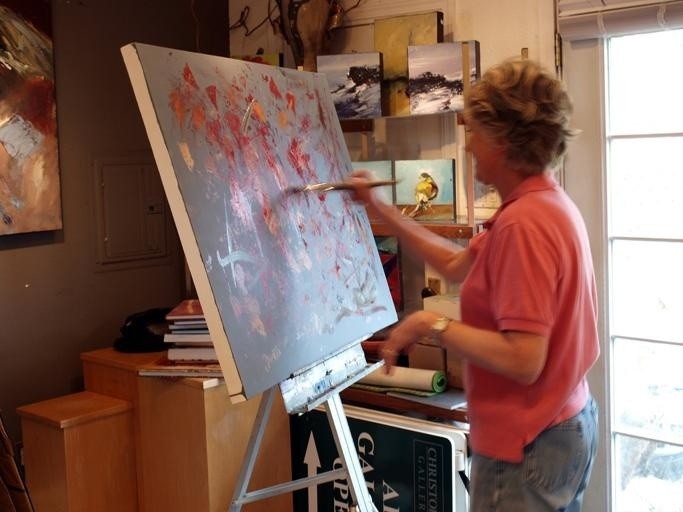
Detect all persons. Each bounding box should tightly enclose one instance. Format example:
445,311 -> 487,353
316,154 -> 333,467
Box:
350,59 -> 600,512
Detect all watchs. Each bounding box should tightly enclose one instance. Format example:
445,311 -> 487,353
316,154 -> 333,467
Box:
430,317 -> 452,343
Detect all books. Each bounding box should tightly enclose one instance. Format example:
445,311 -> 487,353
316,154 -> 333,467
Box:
384,390 -> 468,410
139,297 -> 224,378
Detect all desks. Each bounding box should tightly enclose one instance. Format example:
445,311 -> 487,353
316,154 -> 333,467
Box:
341,388 -> 468,424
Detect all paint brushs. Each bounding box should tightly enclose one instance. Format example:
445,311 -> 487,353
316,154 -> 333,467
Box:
293,179 -> 403,192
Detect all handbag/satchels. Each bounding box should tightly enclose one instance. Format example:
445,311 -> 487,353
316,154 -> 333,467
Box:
112,305 -> 175,353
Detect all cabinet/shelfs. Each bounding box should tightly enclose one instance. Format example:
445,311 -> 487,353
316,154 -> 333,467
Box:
17,347 -> 292,512
